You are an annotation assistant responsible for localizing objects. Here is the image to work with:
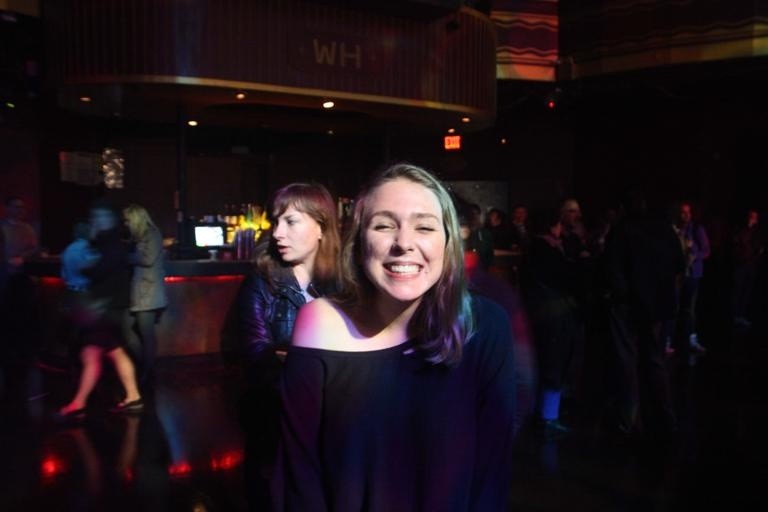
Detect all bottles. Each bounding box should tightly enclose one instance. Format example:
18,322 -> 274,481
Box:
222,202 -> 253,225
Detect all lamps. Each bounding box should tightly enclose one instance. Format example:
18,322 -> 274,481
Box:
444,128 -> 461,150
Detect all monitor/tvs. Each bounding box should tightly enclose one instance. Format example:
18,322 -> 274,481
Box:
192,222 -> 227,249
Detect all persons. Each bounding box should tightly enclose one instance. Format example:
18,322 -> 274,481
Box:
0,194 -> 41,280
217,181 -> 343,512
121,203 -> 175,372
49,201 -> 145,420
445,185 -> 767,449
274,159 -> 532,509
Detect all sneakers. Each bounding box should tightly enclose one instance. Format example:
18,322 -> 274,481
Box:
110,397 -> 145,414
58,402 -> 88,418
